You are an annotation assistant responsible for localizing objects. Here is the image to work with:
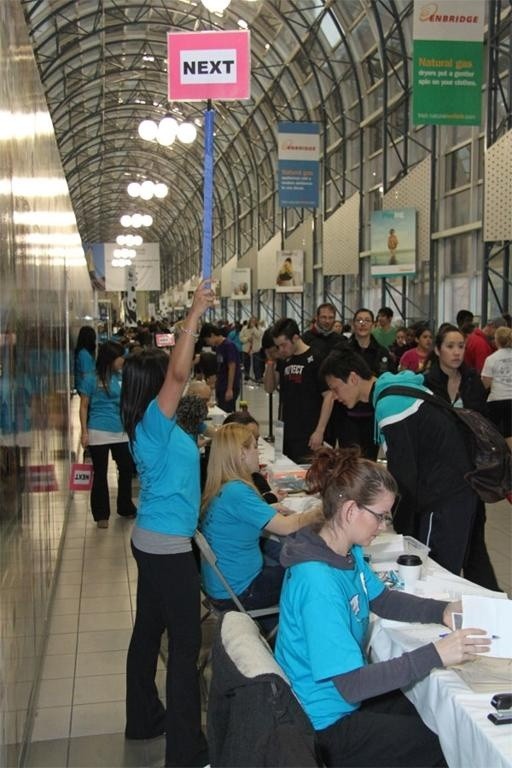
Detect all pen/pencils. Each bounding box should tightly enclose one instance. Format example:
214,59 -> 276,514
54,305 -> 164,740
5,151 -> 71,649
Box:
439,633 -> 497,639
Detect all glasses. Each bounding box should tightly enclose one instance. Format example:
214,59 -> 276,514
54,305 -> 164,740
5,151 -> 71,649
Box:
354,319 -> 373,323
355,500 -> 395,527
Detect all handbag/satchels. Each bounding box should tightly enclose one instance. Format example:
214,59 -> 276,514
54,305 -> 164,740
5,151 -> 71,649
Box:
69,462 -> 95,491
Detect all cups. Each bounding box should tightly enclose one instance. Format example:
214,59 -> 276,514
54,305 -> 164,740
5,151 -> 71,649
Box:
397,554 -> 423,584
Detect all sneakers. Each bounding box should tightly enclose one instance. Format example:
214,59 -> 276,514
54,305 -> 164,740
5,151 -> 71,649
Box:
96,518 -> 111,529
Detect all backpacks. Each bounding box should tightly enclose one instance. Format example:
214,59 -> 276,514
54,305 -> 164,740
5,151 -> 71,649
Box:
374,375 -> 511,507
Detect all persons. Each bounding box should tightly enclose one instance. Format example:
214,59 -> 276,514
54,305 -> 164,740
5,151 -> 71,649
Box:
0,305 -> 74,533
280,257 -> 296,286
387,229 -> 398,261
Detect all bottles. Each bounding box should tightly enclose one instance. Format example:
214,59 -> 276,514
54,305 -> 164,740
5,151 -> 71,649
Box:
239,401 -> 249,413
376,458 -> 388,471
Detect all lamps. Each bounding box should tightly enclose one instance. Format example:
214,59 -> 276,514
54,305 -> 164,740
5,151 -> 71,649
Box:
115,206 -> 156,230
134,108 -> 206,147
123,171 -> 171,203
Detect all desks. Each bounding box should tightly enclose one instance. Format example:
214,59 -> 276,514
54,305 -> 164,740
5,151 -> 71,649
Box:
206,407 -> 510,766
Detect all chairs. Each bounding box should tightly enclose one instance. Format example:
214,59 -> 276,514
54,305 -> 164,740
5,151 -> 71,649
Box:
215,602 -> 331,768
184,527 -> 287,686
